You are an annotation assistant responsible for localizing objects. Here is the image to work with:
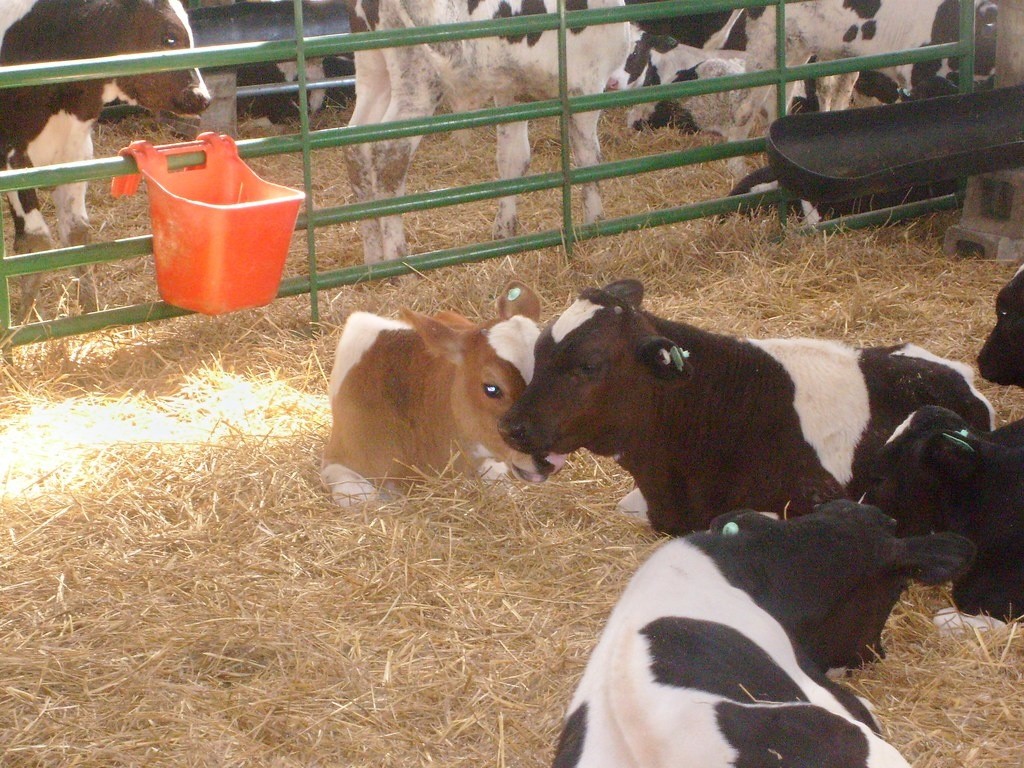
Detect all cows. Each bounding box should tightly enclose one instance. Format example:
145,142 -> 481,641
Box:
0,0 -> 1024,768
